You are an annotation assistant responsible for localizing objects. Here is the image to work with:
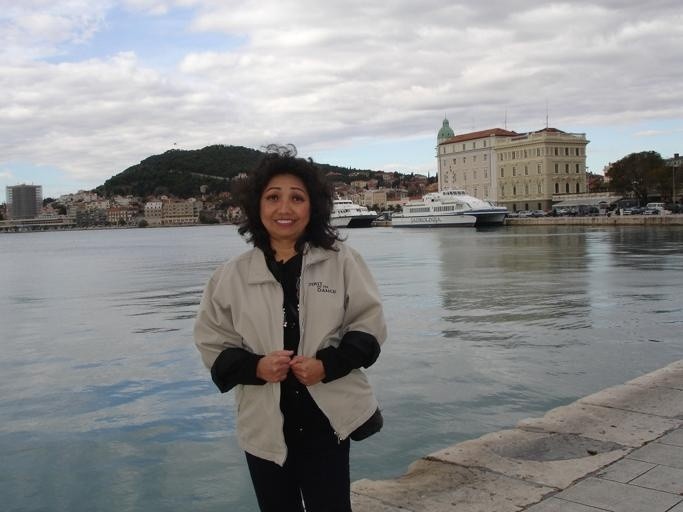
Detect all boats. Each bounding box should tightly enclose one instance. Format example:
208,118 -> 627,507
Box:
389,189 -> 507,227
327,195 -> 378,229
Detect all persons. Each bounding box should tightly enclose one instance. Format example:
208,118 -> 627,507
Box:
193,142 -> 388,512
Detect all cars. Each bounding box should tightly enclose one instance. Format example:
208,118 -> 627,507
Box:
506,201 -> 664,216
